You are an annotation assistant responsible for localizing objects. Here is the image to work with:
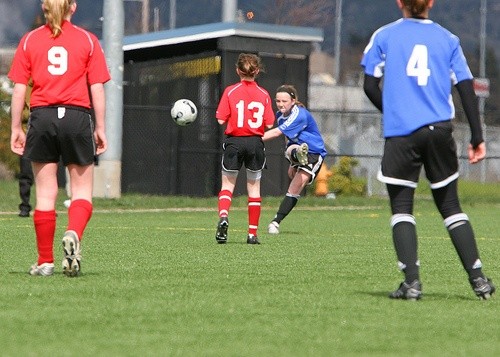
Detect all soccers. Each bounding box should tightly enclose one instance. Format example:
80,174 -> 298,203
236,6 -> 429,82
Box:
171,99 -> 198,127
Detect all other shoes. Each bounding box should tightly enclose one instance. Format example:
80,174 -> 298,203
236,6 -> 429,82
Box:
19,210 -> 29,217
28,262 -> 55,276
61,230 -> 81,277
215,216 -> 228,243
268,221 -> 280,234
247,233 -> 261,245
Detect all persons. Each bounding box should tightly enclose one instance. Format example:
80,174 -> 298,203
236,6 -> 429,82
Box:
359,0 -> 496,300
215,53 -> 275,245
260,83 -> 327,233
9,0 -> 111,277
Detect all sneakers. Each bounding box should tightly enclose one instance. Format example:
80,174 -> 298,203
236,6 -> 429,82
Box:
388,280 -> 422,300
470,276 -> 496,300
296,143 -> 309,166
